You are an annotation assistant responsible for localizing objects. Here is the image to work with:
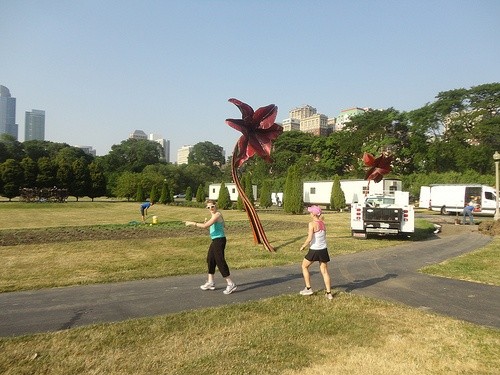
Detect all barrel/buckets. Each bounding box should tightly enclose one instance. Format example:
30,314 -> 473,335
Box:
153,215 -> 158,225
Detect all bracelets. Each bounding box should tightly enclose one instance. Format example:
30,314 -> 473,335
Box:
195,222 -> 198,226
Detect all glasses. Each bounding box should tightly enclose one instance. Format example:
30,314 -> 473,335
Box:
207,205 -> 216,210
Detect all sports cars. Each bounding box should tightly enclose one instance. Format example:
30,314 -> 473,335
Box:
173,193 -> 185,198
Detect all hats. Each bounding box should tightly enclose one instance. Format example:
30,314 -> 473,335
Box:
308,205 -> 322,216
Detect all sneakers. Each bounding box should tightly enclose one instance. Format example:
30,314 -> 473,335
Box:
200,282 -> 216,290
325,293 -> 334,300
222,283 -> 238,295
299,287 -> 313,295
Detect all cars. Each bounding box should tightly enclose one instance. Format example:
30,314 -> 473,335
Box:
349,190 -> 415,240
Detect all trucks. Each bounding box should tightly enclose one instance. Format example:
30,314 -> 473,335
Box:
302,177 -> 403,210
208,183 -> 258,202
418,183 -> 500,217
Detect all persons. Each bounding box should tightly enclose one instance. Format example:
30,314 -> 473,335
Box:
300,205 -> 334,300
140,202 -> 155,221
183,199 -> 238,294
461,205 -> 474,225
